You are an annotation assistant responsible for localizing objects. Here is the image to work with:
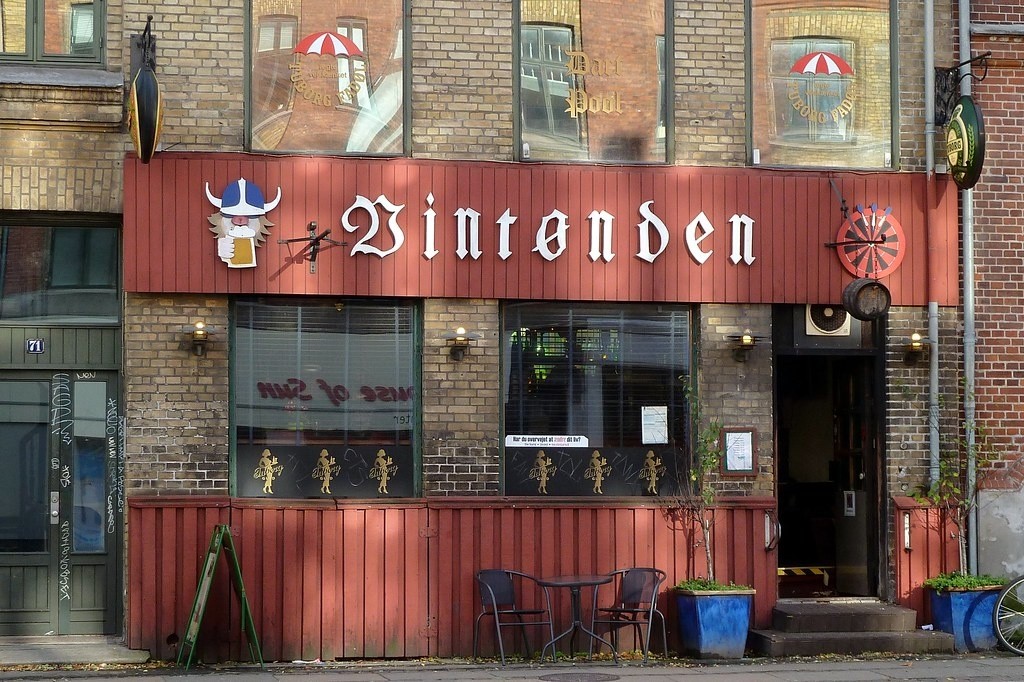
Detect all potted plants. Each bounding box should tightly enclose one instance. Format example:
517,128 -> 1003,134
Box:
892,375 -> 1021,651
652,373 -> 757,660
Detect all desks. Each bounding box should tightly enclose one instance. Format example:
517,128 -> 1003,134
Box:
536,574 -> 619,667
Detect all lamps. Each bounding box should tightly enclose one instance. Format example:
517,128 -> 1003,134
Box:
901,332 -> 937,367
727,329 -> 768,362
443,327 -> 482,361
178,321 -> 216,356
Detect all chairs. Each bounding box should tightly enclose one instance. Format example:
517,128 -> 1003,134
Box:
474,568 -> 557,668
588,566 -> 668,663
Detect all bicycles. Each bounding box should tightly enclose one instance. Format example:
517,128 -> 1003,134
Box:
992,576 -> 1024,658
